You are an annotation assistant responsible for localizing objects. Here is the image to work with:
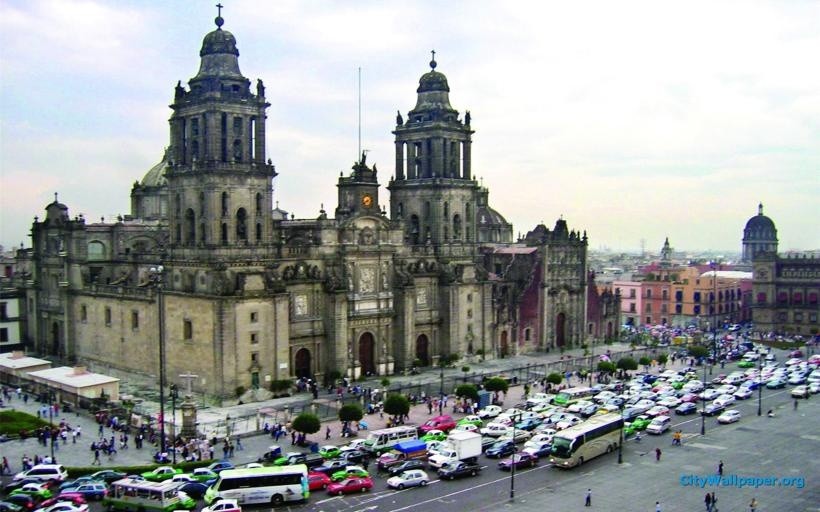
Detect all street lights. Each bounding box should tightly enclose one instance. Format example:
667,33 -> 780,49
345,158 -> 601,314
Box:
705,258 -> 719,367
146,261 -> 171,461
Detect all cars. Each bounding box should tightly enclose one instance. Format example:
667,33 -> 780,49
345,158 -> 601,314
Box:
0,324 -> 819,512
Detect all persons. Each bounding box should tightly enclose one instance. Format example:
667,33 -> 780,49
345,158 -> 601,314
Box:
655,501 -> 660,512
709,492 -> 720,511
704,492 -> 710,510
584,488 -> 592,507
749,498 -> 758,512
0,323 -> 819,474
